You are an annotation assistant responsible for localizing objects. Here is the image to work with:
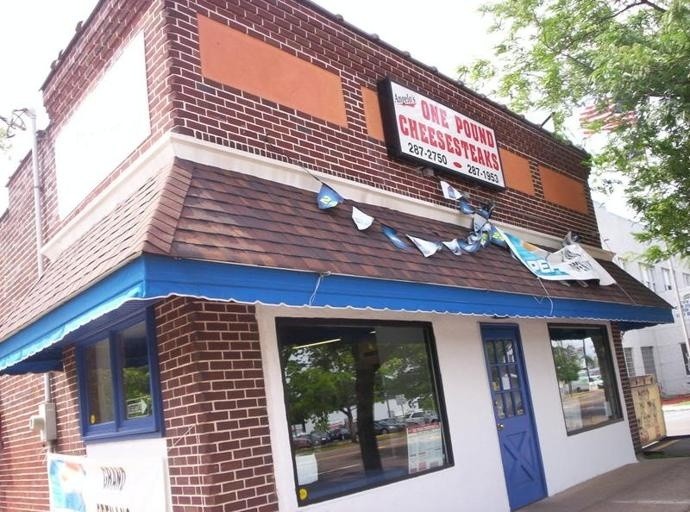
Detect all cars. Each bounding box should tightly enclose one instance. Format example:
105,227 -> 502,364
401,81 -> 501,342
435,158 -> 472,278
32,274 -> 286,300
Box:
565,367 -> 604,392
292,408 -> 439,449
